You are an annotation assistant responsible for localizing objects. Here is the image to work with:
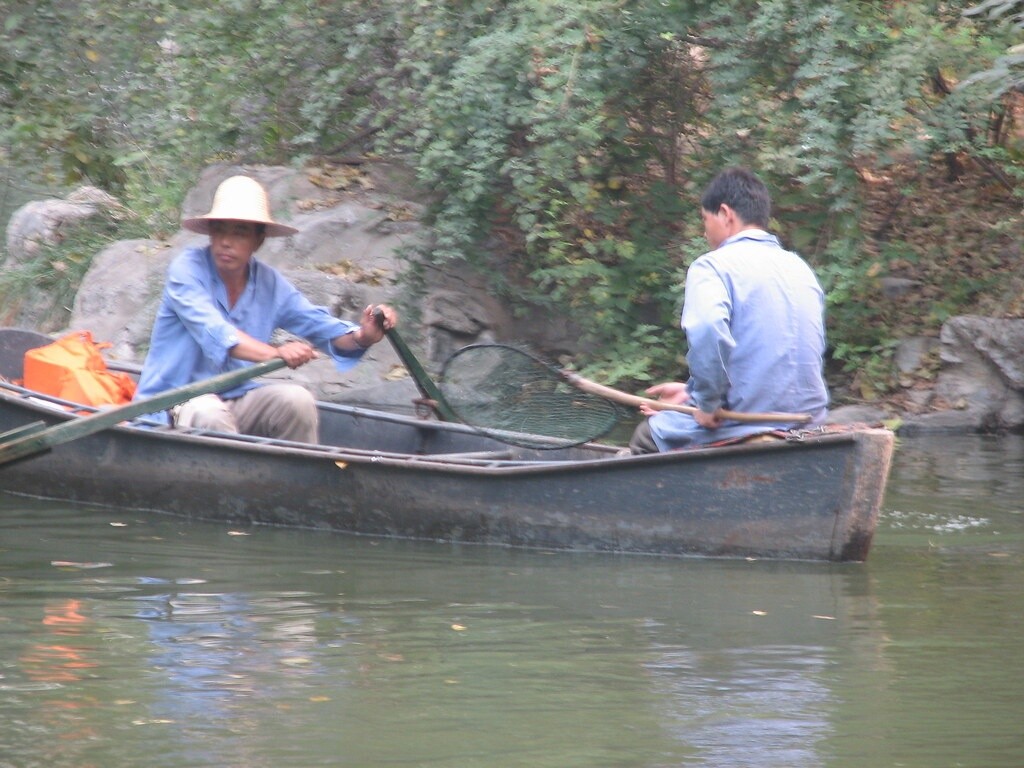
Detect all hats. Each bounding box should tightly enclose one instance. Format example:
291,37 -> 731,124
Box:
182,176 -> 299,237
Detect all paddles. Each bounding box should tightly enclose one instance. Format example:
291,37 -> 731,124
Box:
0,357 -> 288,469
371,308 -> 458,423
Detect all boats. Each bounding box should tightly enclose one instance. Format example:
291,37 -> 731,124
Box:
0,326 -> 897,565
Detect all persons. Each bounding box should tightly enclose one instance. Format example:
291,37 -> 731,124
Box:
131,175 -> 397,446
629,166 -> 828,455
